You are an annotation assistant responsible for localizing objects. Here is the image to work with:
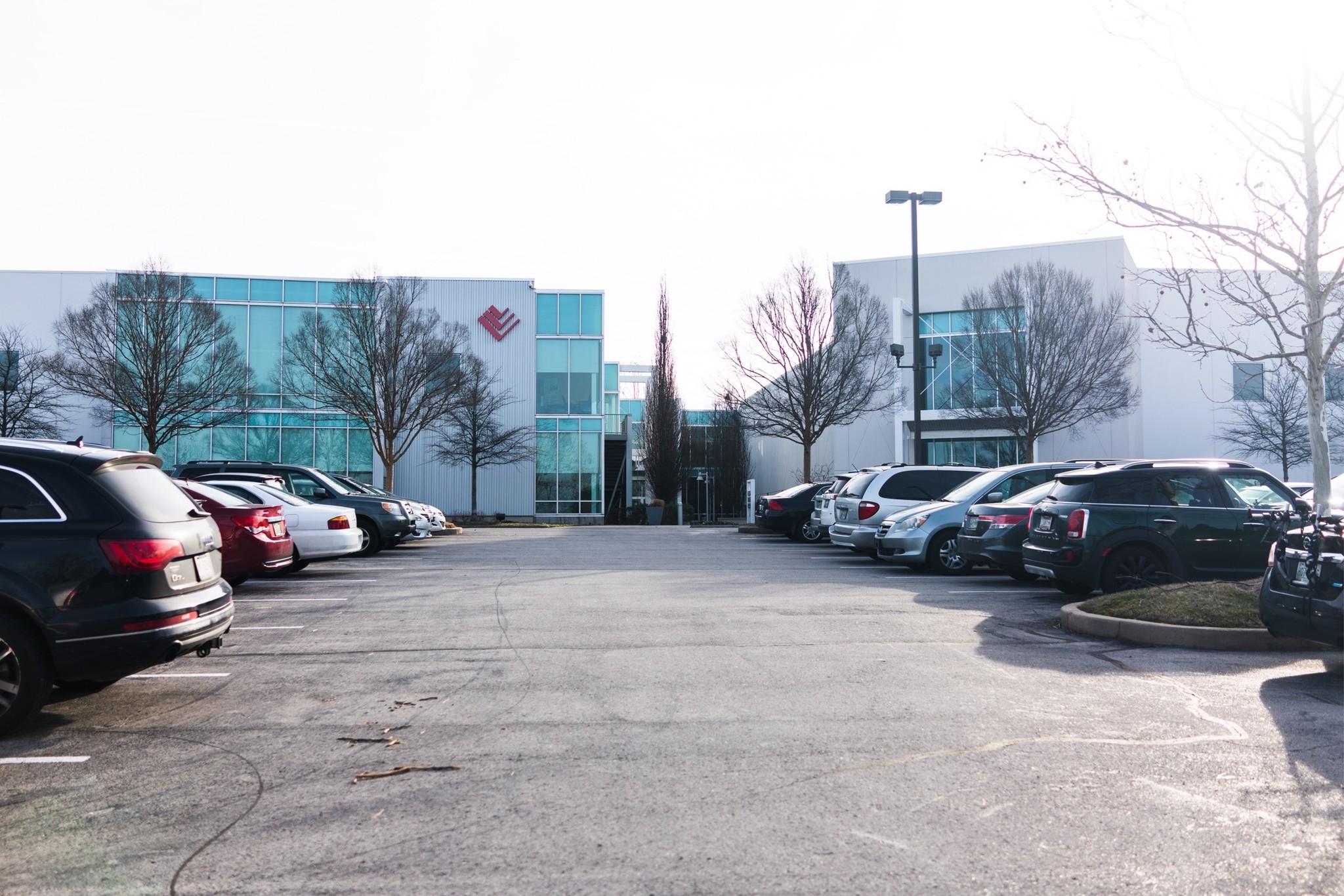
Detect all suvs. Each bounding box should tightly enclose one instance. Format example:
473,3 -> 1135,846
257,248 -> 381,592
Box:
828,461 -> 995,561
0,435 -> 235,737
1021,458 -> 1313,600
809,468 -> 859,539
169,459 -> 416,558
874,457 -> 1120,578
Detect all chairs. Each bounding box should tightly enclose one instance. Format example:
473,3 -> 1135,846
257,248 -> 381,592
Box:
1189,480 -> 1210,506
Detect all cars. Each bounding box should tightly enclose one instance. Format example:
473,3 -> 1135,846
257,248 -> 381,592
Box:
1223,469 -> 1344,519
199,480 -> 363,575
755,481 -> 835,544
956,476 -> 1056,580
292,472 -> 447,548
1257,515 -> 1344,652
170,477 -> 294,588
189,472 -> 289,493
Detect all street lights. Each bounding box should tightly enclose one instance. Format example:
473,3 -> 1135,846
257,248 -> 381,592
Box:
884,190 -> 943,462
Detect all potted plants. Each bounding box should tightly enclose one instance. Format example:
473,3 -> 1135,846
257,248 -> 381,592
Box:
646,498 -> 665,525
